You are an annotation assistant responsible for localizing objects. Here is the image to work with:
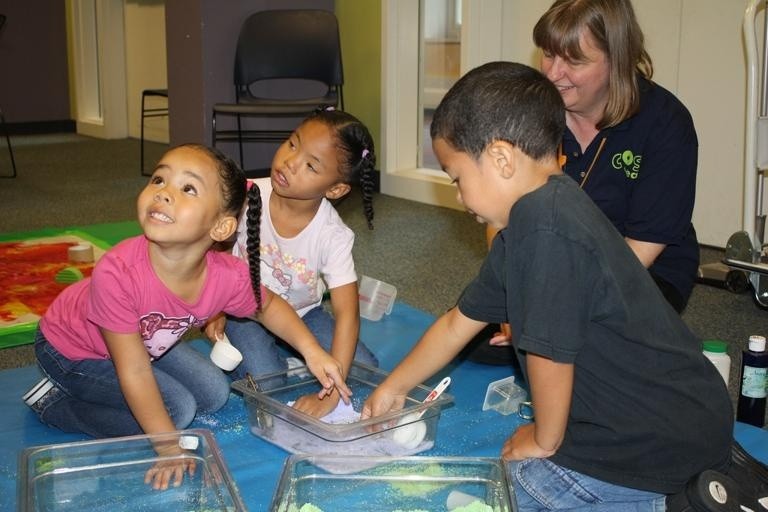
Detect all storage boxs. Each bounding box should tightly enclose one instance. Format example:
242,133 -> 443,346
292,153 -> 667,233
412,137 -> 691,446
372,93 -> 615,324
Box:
268,451 -> 518,512
229,356 -> 455,475
15,428 -> 248,512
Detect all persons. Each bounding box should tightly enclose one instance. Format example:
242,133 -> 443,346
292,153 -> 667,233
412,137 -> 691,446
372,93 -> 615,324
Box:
362,62 -> 768,512
201,105 -> 375,426
454,0 -> 699,366
22,144 -> 353,491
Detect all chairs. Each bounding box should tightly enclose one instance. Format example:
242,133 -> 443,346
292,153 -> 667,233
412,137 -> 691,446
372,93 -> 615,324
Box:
208,6 -> 349,179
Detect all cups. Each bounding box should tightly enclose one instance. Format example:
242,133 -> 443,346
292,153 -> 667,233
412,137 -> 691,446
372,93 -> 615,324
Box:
357,274 -> 398,323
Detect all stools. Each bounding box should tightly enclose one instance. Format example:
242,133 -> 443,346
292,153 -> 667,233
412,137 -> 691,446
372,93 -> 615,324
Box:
137,88 -> 171,177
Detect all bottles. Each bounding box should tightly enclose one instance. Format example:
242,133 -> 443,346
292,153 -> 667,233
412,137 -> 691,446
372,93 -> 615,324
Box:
737,336 -> 768,428
701,340 -> 731,389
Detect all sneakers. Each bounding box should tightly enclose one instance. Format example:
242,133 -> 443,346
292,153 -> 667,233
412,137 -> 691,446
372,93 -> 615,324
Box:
724,439 -> 768,511
685,469 -> 768,512
22,376 -> 72,424
